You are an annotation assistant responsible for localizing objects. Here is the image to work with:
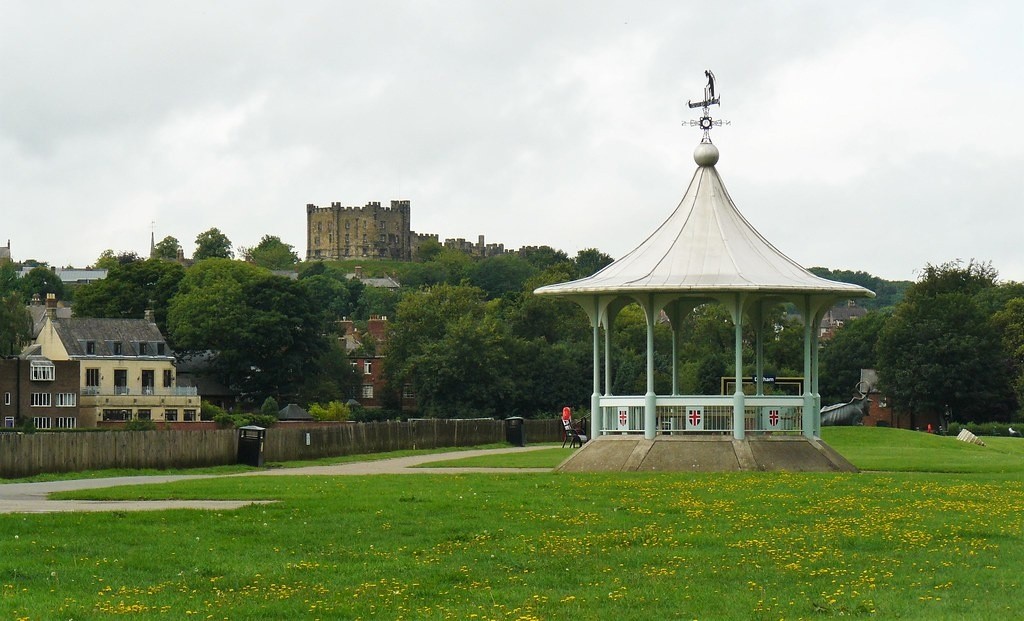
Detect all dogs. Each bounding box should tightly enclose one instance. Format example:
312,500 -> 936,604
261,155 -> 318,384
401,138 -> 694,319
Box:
1009,428 -> 1021,437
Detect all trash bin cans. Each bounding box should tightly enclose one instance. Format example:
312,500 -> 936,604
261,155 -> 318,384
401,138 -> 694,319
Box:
505,417 -> 525,447
876,420 -> 887,426
237,425 -> 265,467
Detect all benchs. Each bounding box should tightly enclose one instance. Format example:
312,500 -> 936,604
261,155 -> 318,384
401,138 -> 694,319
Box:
652,404 -> 755,435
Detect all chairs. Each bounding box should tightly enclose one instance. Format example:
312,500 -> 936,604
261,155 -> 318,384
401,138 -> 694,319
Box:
561,416 -> 586,449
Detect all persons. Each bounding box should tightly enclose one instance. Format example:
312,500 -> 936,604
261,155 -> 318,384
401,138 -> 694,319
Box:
1009,426 -> 1020,436
